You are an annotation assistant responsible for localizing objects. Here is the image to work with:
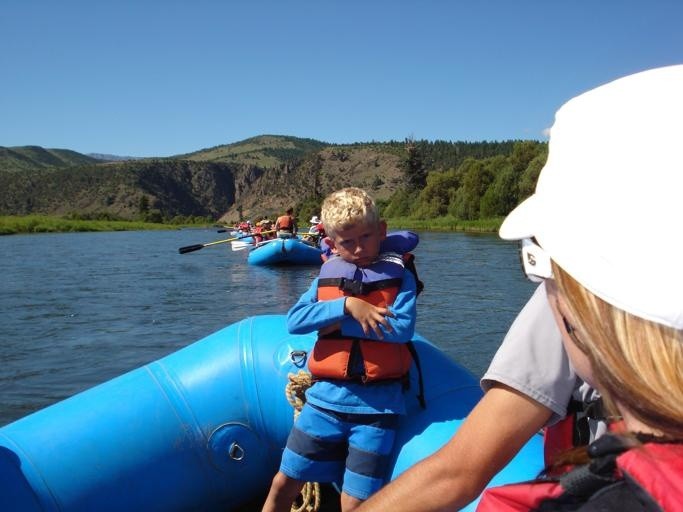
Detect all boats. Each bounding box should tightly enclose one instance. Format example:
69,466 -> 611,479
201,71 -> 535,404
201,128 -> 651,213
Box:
0,315 -> 545,512
230,231 -> 322,266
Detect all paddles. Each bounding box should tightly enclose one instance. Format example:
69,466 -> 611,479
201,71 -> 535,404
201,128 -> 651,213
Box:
217,230 -> 239,233
179,230 -> 277,254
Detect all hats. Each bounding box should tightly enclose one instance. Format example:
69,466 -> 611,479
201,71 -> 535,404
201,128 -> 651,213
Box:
309,216 -> 320,223
497,64 -> 682,329
256,220 -> 272,227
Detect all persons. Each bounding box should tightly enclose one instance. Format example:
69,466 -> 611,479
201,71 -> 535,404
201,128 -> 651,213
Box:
473,63 -> 683,511
230,204 -> 326,249
349,227 -> 623,511
259,186 -> 416,511
